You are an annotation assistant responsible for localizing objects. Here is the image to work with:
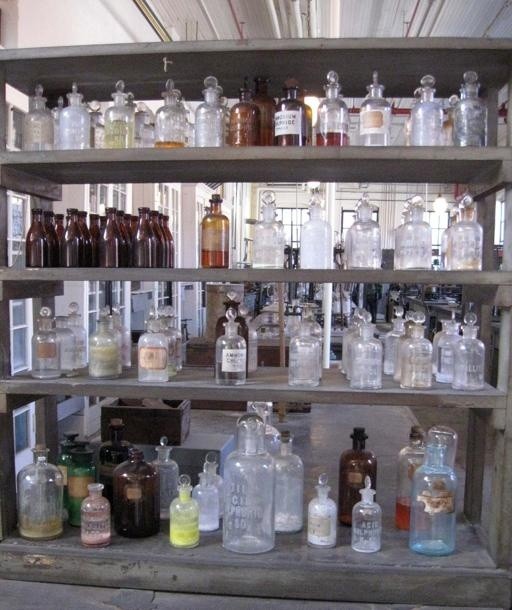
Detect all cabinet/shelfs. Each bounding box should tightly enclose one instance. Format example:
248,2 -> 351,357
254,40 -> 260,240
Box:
4,81 -> 39,504
0,37 -> 511,606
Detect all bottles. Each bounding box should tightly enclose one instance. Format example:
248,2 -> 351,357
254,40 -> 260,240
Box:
113,447 -> 162,539
149,435 -> 181,520
192,470 -> 219,533
98,417 -> 132,520
382,305 -> 433,391
390,195 -> 434,272
438,193 -> 483,272
241,399 -> 281,456
68,440 -> 97,527
57,431 -> 78,508
449,71 -> 489,151
13,443 -> 66,540
349,474 -> 386,555
166,474 -> 201,550
404,73 -> 443,147
296,193 -> 336,269
357,70 -> 392,151
271,428 -> 305,535
202,451 -> 223,521
63,302 -> 87,372
213,290 -> 262,386
344,193 -> 382,269
338,308 -> 383,392
24,204 -> 176,268
29,306 -> 62,380
220,419 -> 276,555
305,472 -> 337,550
80,482 -> 111,548
13,70 -> 352,151
248,189 -> 286,270
394,424 -> 425,530
338,426 -> 377,527
52,315 -> 77,376
411,443 -> 456,557
86,302 -> 185,384
286,302 -> 325,387
432,311 -> 484,392
200,194 -> 230,268
